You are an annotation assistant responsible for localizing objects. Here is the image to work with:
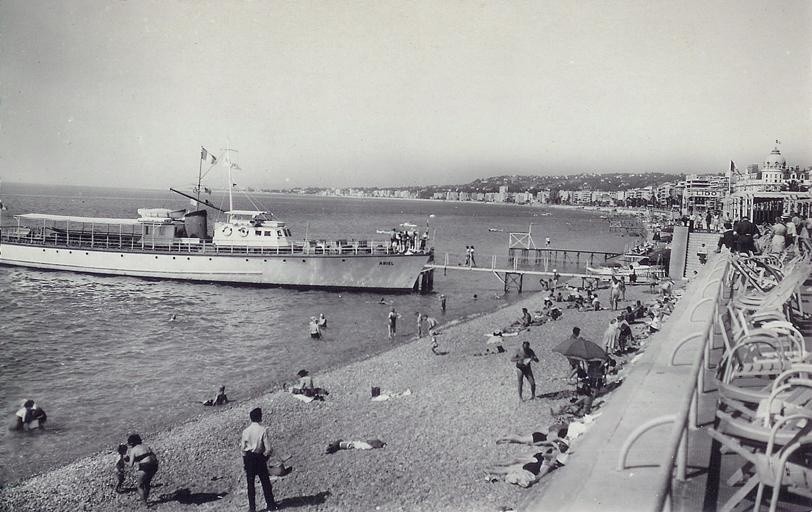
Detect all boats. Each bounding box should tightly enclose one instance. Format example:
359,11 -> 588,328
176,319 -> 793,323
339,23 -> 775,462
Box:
372,227 -> 401,236
1,139 -> 433,293
489,225 -> 504,233
398,221 -> 418,227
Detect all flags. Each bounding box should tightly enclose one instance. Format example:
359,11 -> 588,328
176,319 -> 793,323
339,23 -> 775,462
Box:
201,148 -> 207,161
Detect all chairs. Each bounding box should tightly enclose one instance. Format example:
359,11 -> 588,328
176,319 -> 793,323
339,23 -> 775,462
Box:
708,253 -> 812,512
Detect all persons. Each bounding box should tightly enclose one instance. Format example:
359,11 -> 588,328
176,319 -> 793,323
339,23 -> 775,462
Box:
170,314 -> 176,320
484,386 -> 593,487
485,287 -> 600,400
212,384 -> 236,406
24,409 -> 36,432
15,399 -> 35,433
387,294 -> 446,355
292,369 -> 313,396
603,212 -> 812,355
33,406 -> 48,431
127,433 -> 158,501
465,245 -> 476,267
308,313 -> 326,338
240,407 -> 281,512
111,444 -> 129,495
390,228 -> 428,254
